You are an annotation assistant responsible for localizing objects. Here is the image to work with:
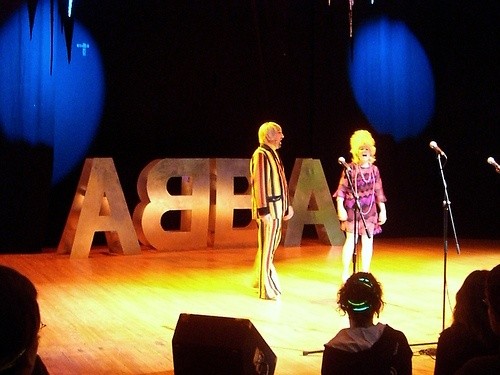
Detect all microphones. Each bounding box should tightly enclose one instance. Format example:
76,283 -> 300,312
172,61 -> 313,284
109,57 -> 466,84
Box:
338,157 -> 352,171
487,157 -> 500,170
429,141 -> 447,159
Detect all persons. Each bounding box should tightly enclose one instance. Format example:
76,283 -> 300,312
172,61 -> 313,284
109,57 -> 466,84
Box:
435,270 -> 500,375
0,264 -> 43,375
486,264 -> 500,335
336,130 -> 387,286
250,122 -> 294,300
320,272 -> 414,375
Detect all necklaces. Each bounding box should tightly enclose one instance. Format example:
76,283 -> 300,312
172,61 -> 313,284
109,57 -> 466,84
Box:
359,163 -> 372,183
355,163 -> 375,214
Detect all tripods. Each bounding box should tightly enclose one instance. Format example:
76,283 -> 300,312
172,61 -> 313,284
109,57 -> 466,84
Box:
408,153 -> 461,346
302,167 -> 370,355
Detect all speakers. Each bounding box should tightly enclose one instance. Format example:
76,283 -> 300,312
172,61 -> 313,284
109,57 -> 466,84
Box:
172,314 -> 277,375
